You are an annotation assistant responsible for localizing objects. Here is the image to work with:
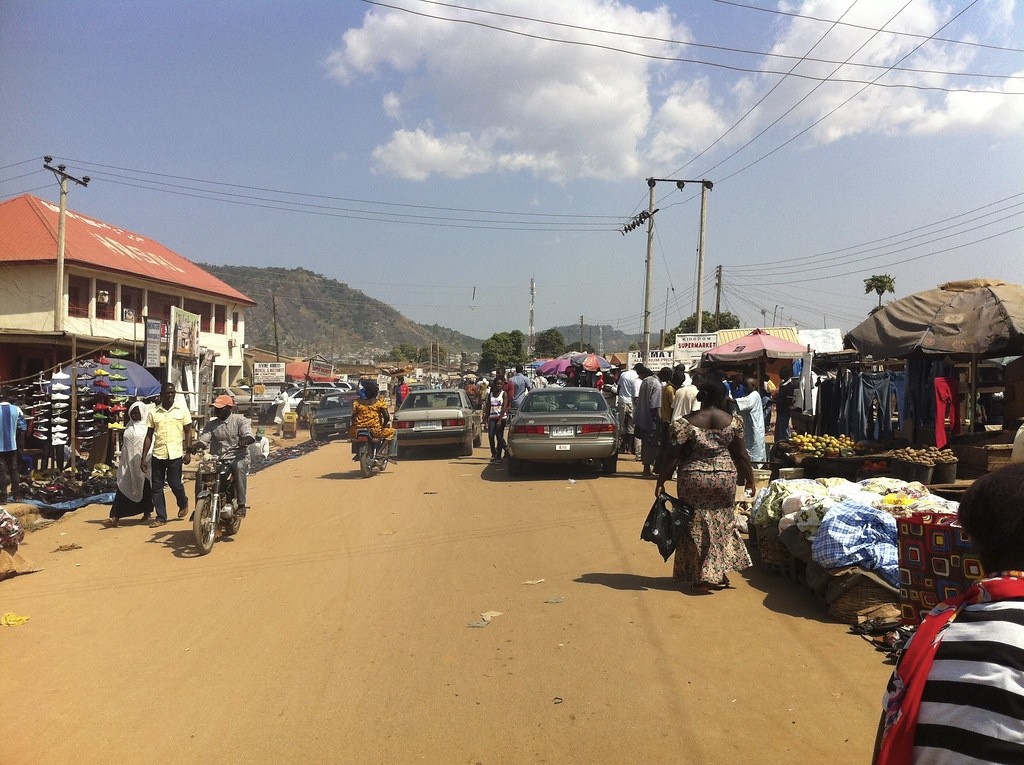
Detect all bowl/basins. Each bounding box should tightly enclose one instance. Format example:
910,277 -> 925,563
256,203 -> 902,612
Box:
841,448 -> 856,457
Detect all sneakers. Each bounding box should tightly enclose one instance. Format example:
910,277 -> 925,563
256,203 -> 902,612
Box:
108,350 -> 129,430
33,378 -> 51,440
76,360 -> 97,452
93,356 -> 111,437
51,373 -> 70,445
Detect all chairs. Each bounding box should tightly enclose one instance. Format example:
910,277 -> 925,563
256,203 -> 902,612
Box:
578,400 -> 598,411
415,399 -> 428,408
532,402 -> 550,412
447,397 -> 458,407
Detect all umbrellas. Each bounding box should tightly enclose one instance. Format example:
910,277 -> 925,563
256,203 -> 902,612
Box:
40,355 -> 162,425
702,329 -> 813,394
844,278 -> 1024,432
527,350 -> 611,387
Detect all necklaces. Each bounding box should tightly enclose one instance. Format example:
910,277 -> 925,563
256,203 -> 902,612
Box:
186,395 -> 254,522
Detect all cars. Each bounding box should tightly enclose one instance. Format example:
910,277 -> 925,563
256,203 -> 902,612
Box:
288,382 -> 362,392
507,387 -> 621,475
213,387 -> 251,415
392,389 -> 482,456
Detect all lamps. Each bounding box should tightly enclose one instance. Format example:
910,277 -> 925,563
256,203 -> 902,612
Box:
241,343 -> 249,348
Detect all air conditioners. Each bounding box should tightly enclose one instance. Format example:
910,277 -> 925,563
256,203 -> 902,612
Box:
228,338 -> 238,347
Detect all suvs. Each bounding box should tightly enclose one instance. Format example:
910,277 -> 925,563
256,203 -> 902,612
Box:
310,391 -> 359,440
285,387 -> 344,414
405,383 -> 427,391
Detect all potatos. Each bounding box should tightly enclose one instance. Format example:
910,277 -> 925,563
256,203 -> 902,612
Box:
893,444 -> 958,465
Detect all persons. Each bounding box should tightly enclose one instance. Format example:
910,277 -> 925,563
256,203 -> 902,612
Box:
249,427 -> 269,462
348,363 -> 795,479
655,378 -> 756,595
140,383 -> 192,528
103,401 -> 155,526
870,460 -> 1024,765
0,389 -> 79,505
272,384 -> 290,436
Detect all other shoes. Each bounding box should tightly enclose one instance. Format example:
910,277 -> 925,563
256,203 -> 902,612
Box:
494,450 -> 500,462
236,505 -> 246,515
101,517 -> 119,528
272,433 -> 280,436
0,384 -> 34,419
351,453 -> 359,461
503,450 -> 510,458
690,576 -> 730,593
0,498 -> 22,506
141,515 -> 150,521
489,452 -> 496,462
641,472 -> 659,480
387,457 -> 399,464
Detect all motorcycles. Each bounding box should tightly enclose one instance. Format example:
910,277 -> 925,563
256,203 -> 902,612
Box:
193,445 -> 250,555
355,413 -> 398,477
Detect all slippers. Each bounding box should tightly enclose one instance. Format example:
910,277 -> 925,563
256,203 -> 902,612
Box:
149,521 -> 167,528
178,505 -> 188,518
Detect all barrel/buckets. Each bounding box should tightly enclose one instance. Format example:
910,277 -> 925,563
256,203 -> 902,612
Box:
890,457 -> 936,485
933,458 -> 960,484
778,467 -> 805,480
751,469 -> 772,489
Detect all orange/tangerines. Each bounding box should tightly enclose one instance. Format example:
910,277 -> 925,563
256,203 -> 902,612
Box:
790,431 -> 855,455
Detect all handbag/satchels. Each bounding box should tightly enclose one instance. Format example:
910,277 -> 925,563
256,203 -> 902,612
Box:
641,492 -> 694,563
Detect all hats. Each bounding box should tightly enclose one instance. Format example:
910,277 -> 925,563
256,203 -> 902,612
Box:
209,394 -> 234,408
256,426 -> 265,437
596,371 -> 602,376
